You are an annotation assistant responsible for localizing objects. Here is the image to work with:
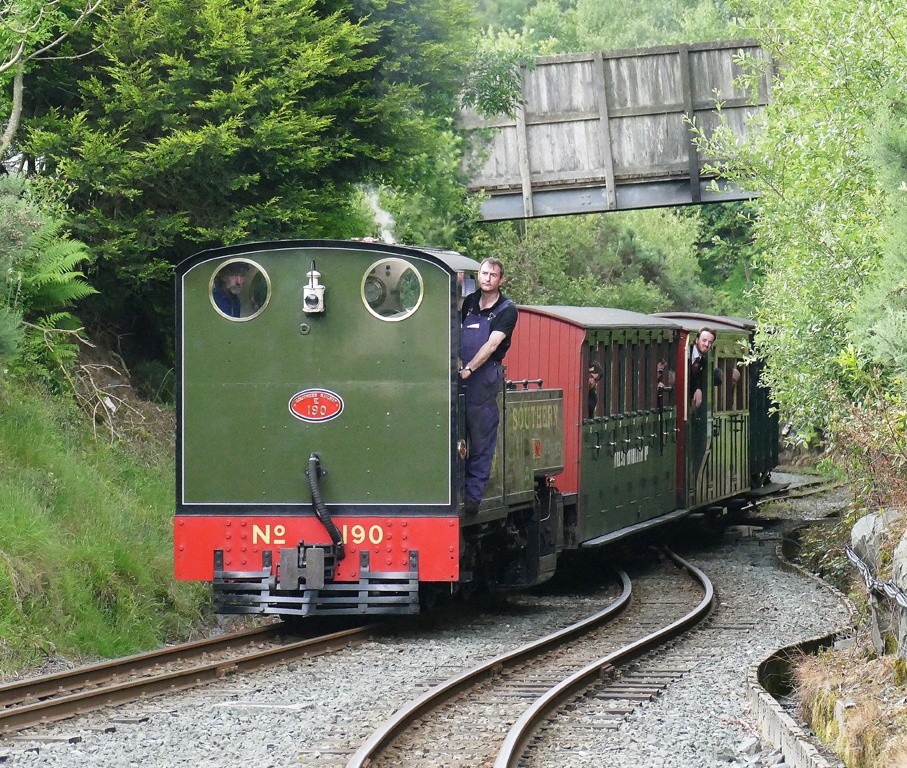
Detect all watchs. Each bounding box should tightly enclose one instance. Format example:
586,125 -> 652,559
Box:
464,366 -> 473,374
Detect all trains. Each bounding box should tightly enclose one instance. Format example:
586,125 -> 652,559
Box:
173,241 -> 781,618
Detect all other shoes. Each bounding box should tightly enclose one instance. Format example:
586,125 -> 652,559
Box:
465,503 -> 478,514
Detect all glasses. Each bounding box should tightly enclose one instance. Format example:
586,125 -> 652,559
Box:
658,369 -> 663,374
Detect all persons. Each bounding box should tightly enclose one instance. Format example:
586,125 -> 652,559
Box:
213,262 -> 249,318
733,368 -> 740,385
457,258 -> 518,514
588,360 -> 603,419
687,327 -> 717,408
656,357 -> 673,407
